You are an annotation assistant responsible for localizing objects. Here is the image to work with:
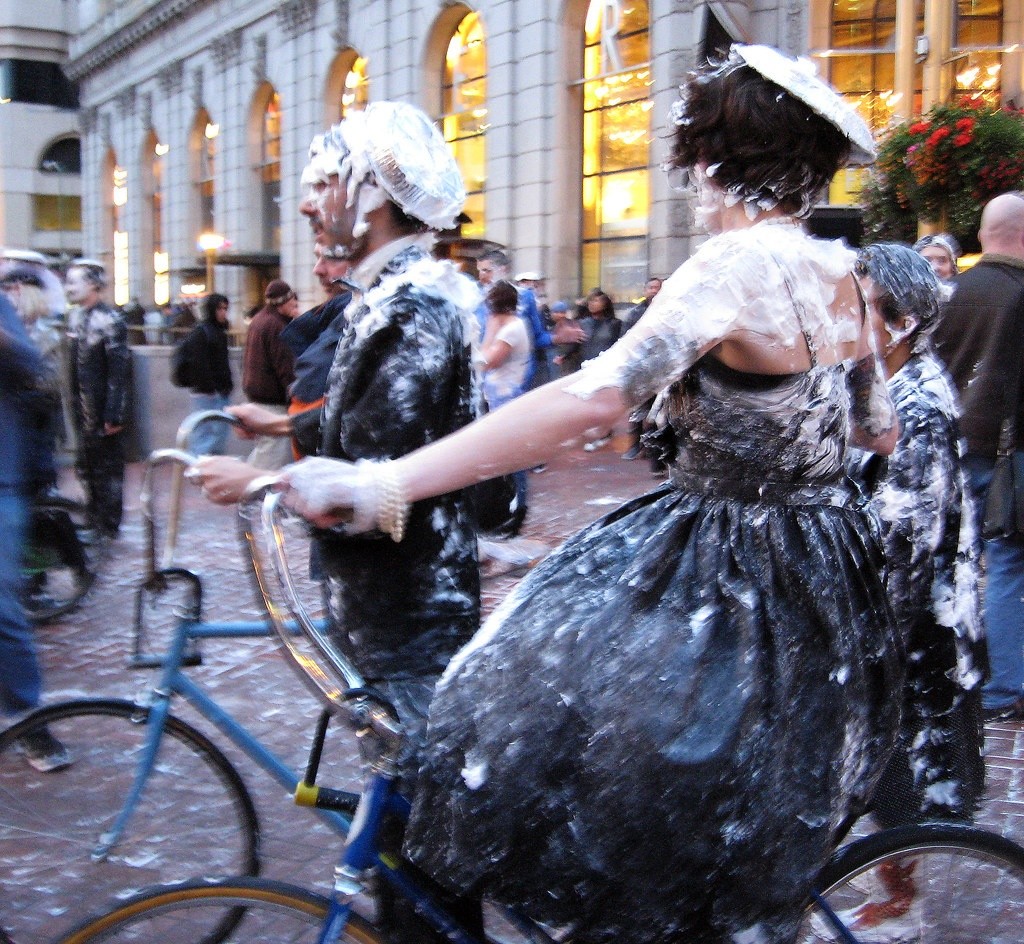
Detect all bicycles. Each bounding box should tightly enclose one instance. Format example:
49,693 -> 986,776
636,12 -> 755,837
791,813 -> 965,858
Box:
53,472 -> 1024,944
0,409 -> 365,944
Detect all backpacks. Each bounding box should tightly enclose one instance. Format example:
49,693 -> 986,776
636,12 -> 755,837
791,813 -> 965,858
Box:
169,323 -> 210,388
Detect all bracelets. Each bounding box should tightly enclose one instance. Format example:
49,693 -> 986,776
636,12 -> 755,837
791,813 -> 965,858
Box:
367,450 -> 411,546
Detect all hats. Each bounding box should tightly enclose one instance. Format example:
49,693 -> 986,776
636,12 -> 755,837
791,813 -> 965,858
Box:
264,279 -> 293,306
324,99 -> 463,228
737,41 -> 878,168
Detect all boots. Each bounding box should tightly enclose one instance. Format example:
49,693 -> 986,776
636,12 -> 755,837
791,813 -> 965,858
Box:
812,852 -> 924,943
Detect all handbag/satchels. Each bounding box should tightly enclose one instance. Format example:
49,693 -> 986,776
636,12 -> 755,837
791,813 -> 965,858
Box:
473,400 -> 526,535
979,414 -> 1024,544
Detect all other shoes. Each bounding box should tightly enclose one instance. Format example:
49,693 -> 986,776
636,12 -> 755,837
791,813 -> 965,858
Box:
583,435 -> 611,452
14,729 -> 70,772
623,443 -> 645,459
981,702 -> 1019,723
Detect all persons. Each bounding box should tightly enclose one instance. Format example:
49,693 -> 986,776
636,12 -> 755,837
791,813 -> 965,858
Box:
121,296 -> 195,346
443,245 -> 666,538
181,98 -> 477,767
267,42 -> 909,944
913,233 -> 963,280
167,241 -> 353,490
838,240 -> 987,944
932,195 -> 1024,721
0,255 -> 136,773
